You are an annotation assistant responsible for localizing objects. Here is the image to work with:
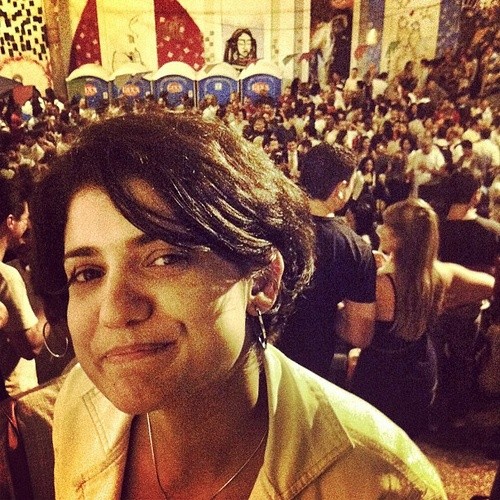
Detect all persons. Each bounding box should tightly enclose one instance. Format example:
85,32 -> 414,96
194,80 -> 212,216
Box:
30,113 -> 448,500
0,7 -> 500,447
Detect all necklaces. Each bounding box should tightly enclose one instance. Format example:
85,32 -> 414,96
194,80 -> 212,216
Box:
146,414 -> 268,500
